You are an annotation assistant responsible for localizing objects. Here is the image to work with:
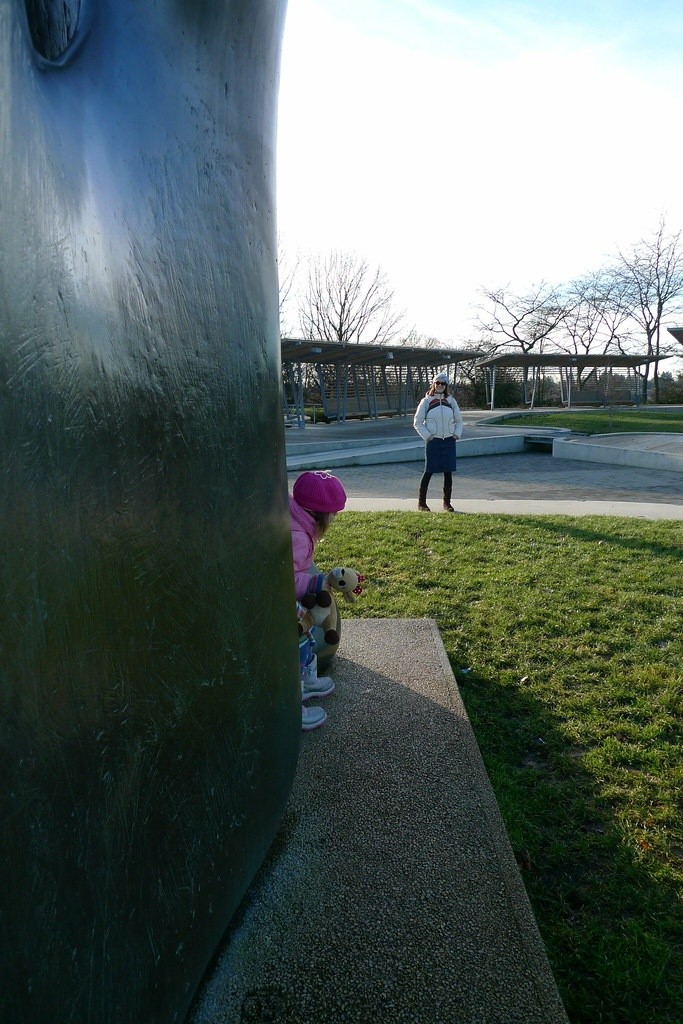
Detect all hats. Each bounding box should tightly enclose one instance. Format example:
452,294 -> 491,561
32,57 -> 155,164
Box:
433,372 -> 449,385
293,470 -> 347,512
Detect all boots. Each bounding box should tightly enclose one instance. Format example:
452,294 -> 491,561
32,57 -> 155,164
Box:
442,487 -> 454,512
418,488 -> 430,512
303,653 -> 335,700
301,680 -> 328,731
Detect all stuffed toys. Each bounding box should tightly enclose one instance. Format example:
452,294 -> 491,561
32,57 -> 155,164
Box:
298,566 -> 361,645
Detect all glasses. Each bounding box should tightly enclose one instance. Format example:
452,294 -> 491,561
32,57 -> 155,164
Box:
434,381 -> 446,386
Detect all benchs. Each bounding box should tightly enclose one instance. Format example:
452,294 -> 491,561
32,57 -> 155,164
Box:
607,390 -> 635,402
323,395 -> 417,419
561,391 -> 602,403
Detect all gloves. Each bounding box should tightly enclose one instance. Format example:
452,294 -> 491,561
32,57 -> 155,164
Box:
309,573 -> 332,594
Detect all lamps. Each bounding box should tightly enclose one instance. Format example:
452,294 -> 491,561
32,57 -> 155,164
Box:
641,359 -> 648,361
303,346 -> 322,353
568,357 -> 577,362
385,348 -> 394,359
440,355 -> 451,359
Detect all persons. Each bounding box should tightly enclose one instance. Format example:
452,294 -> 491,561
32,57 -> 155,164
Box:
413,373 -> 462,512
287,472 -> 347,728
